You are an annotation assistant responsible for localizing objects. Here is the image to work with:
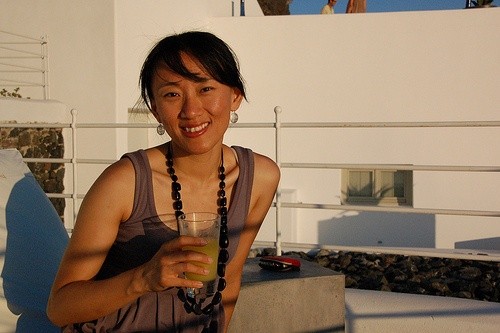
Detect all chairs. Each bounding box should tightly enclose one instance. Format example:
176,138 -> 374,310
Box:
0,148 -> 70,333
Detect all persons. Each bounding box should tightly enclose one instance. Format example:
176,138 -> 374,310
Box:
46,32 -> 281,333
346,0 -> 367,13
321,0 -> 336,14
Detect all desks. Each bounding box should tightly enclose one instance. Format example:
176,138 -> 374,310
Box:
226,256 -> 346,333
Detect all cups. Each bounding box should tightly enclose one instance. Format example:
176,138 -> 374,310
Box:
178,212 -> 221,300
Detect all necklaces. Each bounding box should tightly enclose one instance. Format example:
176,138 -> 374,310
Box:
165,140 -> 229,313
